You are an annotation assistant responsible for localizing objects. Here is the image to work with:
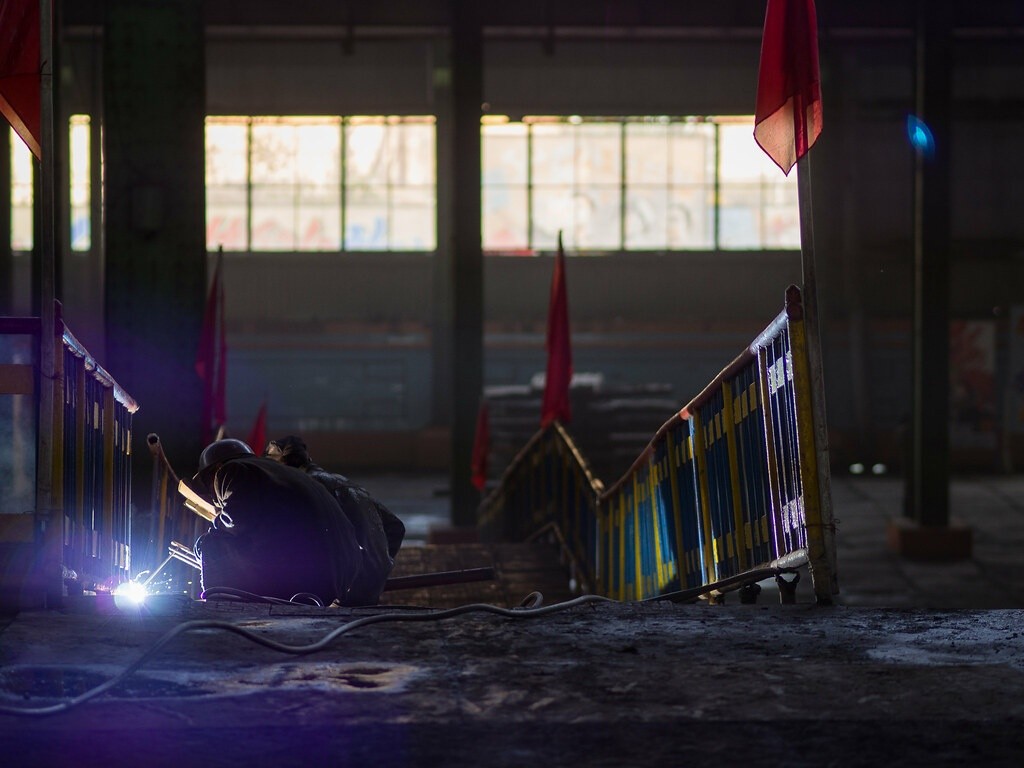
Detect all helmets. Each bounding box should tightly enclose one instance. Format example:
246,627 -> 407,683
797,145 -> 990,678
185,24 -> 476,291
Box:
192,438 -> 256,482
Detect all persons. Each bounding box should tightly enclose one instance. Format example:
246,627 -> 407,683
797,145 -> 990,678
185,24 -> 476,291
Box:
190,439 -> 376,604
263,436 -> 405,606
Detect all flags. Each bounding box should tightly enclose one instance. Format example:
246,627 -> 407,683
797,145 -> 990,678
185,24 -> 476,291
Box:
193,262 -> 225,451
0,0 -> 40,164
540,249 -> 573,430
245,405 -> 265,457
469,397 -> 490,492
753,0 -> 824,176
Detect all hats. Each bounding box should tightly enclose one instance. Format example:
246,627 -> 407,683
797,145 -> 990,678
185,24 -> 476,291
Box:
261,435 -> 309,461
